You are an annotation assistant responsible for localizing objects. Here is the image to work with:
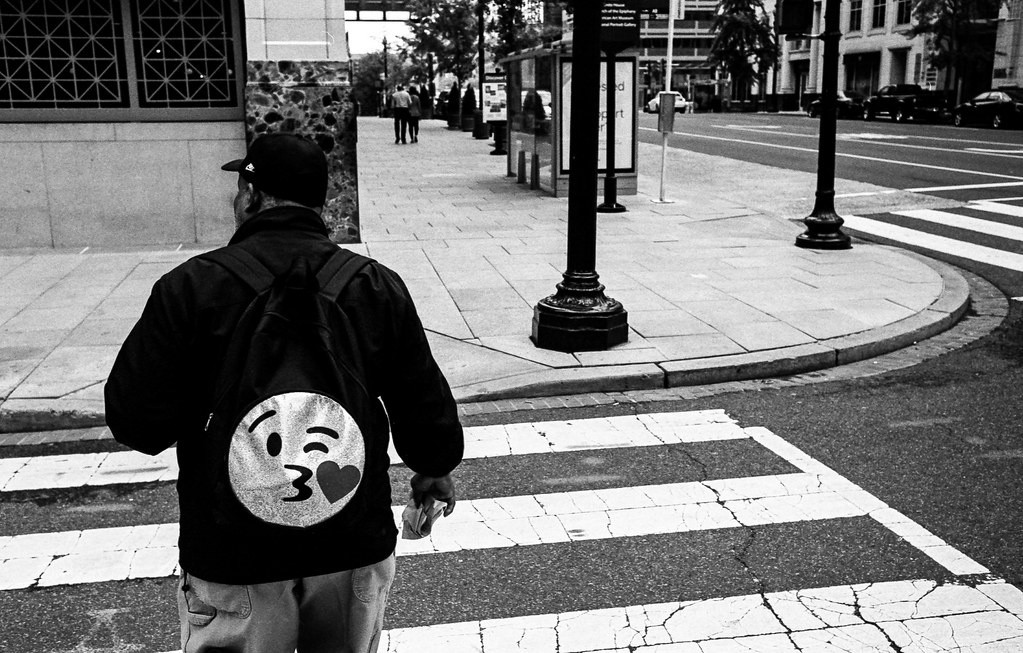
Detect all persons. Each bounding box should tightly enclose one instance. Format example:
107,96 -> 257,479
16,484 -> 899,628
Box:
103,130 -> 464,653
392,83 -> 413,144
859,81 -> 869,97
523,88 -> 546,135
408,86 -> 422,143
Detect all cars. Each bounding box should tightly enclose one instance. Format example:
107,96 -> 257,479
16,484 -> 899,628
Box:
952,89 -> 1023,130
644,91 -> 686,113
807,89 -> 865,120
520,89 -> 551,119
432,89 -> 451,119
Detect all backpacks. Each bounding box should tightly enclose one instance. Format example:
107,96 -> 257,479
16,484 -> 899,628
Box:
194,245 -> 389,530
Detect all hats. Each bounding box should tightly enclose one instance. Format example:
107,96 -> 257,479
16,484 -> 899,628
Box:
222,132 -> 328,207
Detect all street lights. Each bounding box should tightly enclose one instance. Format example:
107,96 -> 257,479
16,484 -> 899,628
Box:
381,36 -> 389,117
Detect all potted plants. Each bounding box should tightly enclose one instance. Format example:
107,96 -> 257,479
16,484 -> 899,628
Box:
446,82 -> 459,128
419,85 -> 432,119
461,84 -> 477,132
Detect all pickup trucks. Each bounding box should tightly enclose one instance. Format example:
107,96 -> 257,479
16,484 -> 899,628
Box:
859,83 -> 943,123
